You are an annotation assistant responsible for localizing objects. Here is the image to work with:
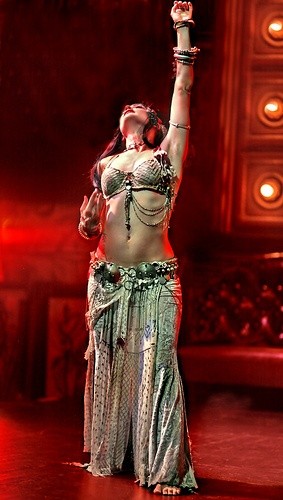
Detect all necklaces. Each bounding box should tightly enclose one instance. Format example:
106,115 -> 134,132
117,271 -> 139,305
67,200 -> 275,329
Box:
123,143 -> 147,151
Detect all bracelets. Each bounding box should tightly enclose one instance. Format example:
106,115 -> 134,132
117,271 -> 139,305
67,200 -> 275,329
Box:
78,223 -> 101,240
173,20 -> 194,28
173,47 -> 200,65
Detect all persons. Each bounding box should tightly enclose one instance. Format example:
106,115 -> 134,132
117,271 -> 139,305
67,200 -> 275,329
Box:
76,0 -> 201,493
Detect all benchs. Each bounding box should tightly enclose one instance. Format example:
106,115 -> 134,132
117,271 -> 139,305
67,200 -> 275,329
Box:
179,250 -> 283,409
